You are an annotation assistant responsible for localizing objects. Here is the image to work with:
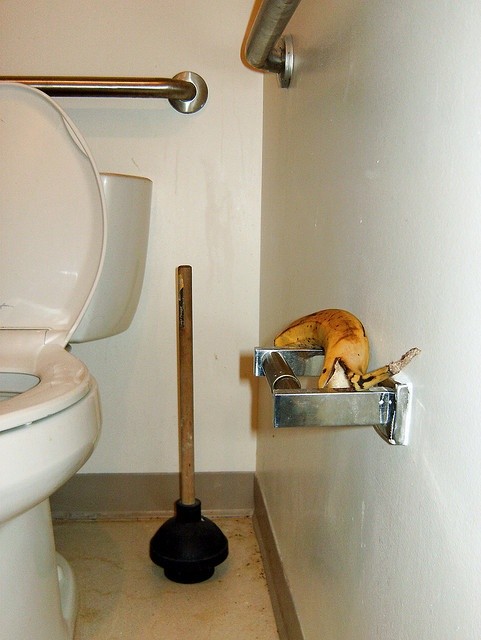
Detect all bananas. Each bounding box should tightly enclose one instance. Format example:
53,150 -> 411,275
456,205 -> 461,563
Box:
272,308 -> 421,392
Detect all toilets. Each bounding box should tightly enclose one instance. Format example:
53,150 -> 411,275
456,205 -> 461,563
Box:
0,81 -> 151,639
